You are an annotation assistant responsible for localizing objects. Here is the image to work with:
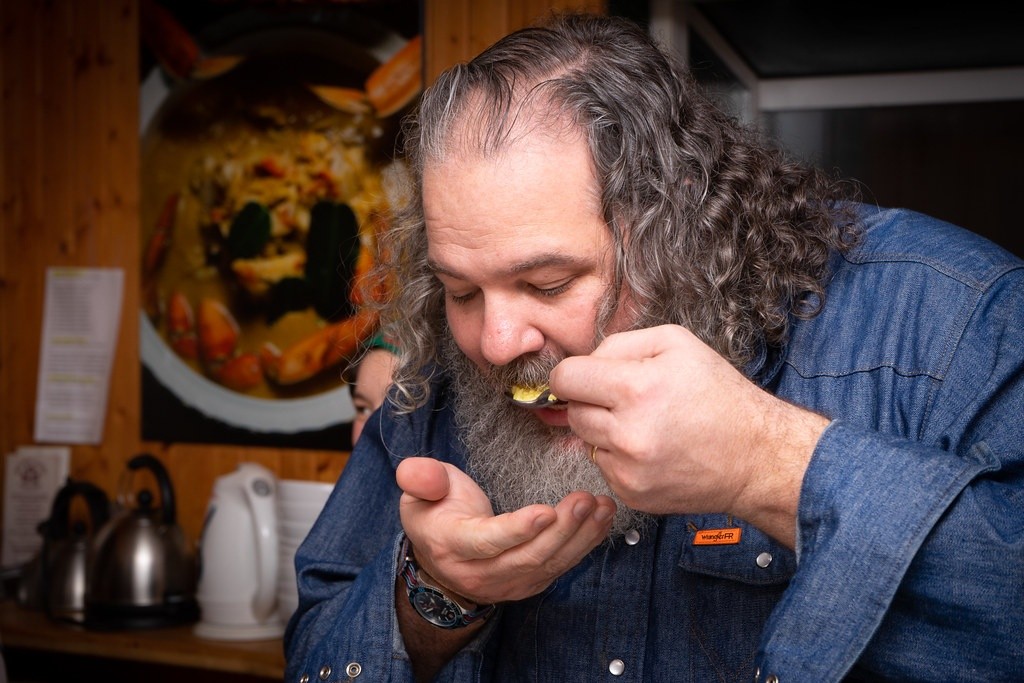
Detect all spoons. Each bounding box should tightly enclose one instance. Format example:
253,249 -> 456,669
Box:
501,387 -> 560,409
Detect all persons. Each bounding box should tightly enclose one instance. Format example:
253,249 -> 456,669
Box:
283,13 -> 1023,682
348,322 -> 408,447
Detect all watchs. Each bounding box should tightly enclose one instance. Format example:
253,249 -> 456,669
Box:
397,537 -> 496,630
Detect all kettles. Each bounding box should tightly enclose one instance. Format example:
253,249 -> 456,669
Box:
93,454 -> 195,628
192,461 -> 286,639
35,476 -> 106,626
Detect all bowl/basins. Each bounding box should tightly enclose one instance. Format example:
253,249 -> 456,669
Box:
141,24 -> 415,435
276,478 -> 337,629
